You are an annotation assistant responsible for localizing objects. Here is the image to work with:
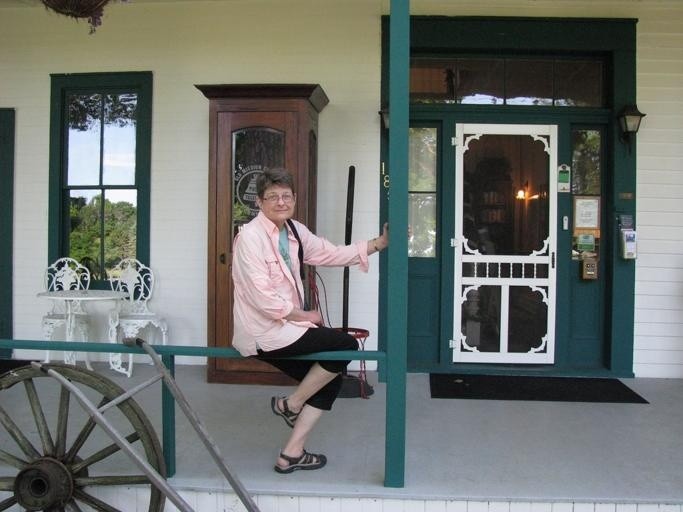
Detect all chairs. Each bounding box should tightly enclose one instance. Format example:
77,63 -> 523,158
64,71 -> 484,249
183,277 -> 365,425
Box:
106,259 -> 168,378
40,257 -> 95,372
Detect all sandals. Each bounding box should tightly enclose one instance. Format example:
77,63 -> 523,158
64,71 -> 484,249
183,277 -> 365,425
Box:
274,449 -> 327,474
270,396 -> 302,428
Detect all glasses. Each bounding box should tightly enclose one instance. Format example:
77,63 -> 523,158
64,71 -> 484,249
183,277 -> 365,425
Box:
262,192 -> 293,205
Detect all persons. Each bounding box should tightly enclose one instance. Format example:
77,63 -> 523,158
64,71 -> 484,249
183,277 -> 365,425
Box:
231,167 -> 390,474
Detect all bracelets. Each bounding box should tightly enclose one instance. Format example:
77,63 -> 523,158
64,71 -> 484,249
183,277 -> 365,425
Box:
373,238 -> 379,251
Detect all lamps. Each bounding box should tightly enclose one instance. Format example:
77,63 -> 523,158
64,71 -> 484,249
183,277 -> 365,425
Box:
616,103 -> 646,144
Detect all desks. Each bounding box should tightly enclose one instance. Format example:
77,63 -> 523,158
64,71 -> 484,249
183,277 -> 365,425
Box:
37,290 -> 130,375
